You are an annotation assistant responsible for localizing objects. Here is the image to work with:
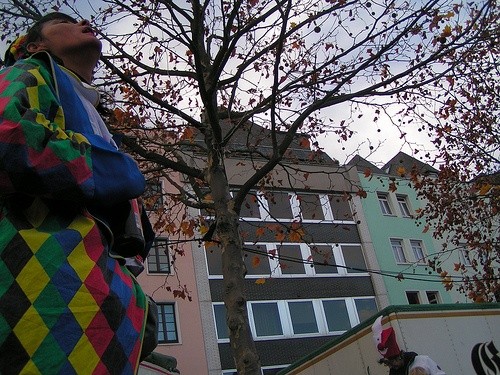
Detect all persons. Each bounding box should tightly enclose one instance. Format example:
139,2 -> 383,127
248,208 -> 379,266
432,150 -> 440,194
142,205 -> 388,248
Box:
0,12 -> 159,375
380,351 -> 449,375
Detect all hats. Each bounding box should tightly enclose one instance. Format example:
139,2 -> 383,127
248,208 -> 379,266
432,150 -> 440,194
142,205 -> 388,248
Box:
372,315 -> 401,359
